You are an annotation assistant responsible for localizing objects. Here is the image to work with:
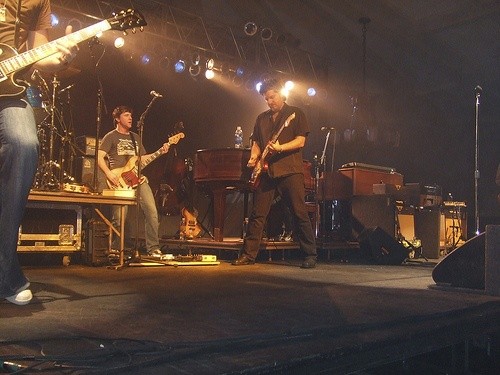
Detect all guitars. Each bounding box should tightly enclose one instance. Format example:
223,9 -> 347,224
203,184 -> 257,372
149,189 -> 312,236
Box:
248,112 -> 296,193
0,8 -> 149,100
176,190 -> 202,239
105,131 -> 185,191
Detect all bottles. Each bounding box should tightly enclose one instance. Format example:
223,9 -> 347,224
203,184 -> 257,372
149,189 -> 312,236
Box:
448,192 -> 453,201
234,126 -> 243,149
242,218 -> 248,238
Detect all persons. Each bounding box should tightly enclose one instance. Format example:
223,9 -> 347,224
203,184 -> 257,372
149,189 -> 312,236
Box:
232,78 -> 320,268
0,1 -> 81,306
98,106 -> 168,259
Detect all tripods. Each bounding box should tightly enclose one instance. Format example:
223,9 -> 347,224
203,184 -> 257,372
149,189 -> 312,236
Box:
116,95 -> 179,270
32,73 -> 76,191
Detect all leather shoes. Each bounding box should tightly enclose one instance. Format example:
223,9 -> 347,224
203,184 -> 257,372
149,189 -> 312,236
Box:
302,258 -> 316,268
232,255 -> 255,265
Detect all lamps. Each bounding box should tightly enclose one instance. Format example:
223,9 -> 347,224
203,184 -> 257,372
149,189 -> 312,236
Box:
332,17 -> 400,148
59,16 -> 329,102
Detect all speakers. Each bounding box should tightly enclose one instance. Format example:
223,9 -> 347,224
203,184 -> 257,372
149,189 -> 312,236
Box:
72,156 -> 109,190
432,233 -> 486,291
86,220 -> 134,266
325,167 -> 467,265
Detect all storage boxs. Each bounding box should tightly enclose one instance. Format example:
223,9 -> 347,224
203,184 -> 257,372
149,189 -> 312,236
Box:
16,203 -> 82,267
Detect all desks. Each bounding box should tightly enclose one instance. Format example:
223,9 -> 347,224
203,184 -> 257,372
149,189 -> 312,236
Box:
28,191 -> 137,270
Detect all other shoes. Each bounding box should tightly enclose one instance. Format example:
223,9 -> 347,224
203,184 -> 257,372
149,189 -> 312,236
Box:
108,249 -> 119,257
148,248 -> 162,258
4,289 -> 32,304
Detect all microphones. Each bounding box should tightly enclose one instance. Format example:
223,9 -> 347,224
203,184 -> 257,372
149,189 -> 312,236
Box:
321,127 -> 335,131
150,91 -> 163,98
58,83 -> 76,94
